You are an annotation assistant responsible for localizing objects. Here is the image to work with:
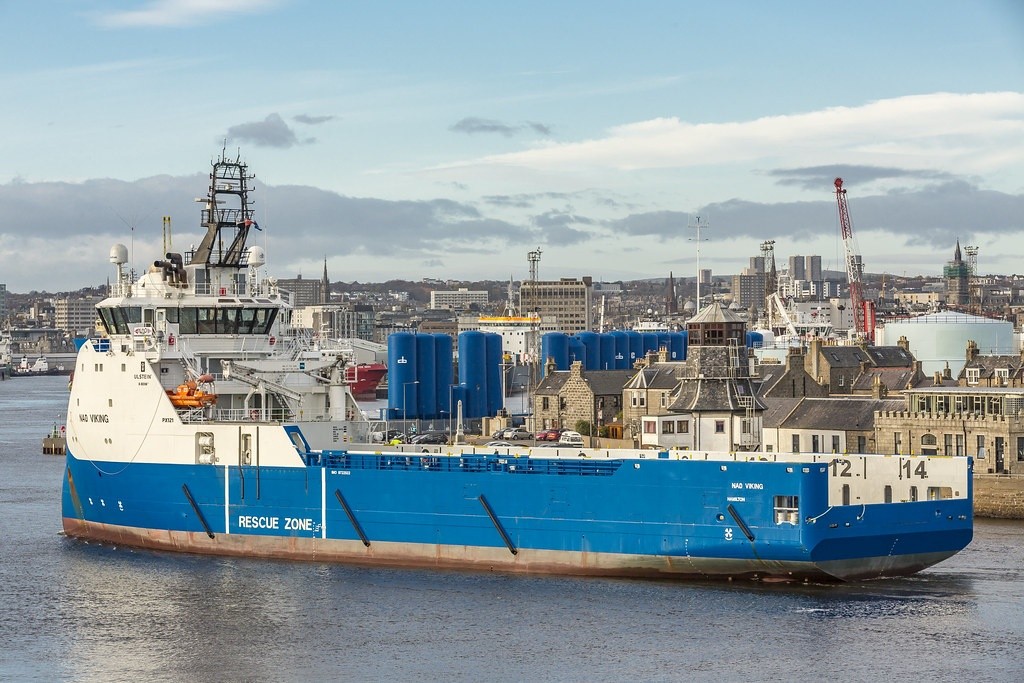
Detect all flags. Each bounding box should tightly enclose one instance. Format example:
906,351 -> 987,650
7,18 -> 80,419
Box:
245,218 -> 262,232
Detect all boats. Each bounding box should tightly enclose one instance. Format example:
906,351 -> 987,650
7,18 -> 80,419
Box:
11,354 -> 65,375
72,336 -> 110,352
874,304 -> 942,327
165,381 -> 220,409
346,361 -> 388,401
61,137 -> 975,584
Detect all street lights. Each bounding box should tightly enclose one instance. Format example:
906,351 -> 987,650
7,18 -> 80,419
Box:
450,383 -> 466,446
838,303 -> 845,330
759,239 -> 776,319
519,382 -> 526,413
538,443 -> 574,448
584,378 -> 592,447
817,306 -> 822,337
403,381 -> 419,440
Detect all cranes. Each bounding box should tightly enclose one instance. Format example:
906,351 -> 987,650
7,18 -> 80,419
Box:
526,247 -> 540,366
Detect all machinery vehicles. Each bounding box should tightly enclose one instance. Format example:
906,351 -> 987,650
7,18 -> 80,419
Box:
832,177 -> 867,344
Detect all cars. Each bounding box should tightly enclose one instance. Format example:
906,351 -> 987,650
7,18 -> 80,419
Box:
492,427 -> 511,440
509,443 -> 529,447
407,433 -> 448,444
382,429 -> 403,438
559,431 -> 584,447
536,430 -> 551,440
484,441 -> 512,447
503,428 -> 533,439
546,426 -> 574,440
